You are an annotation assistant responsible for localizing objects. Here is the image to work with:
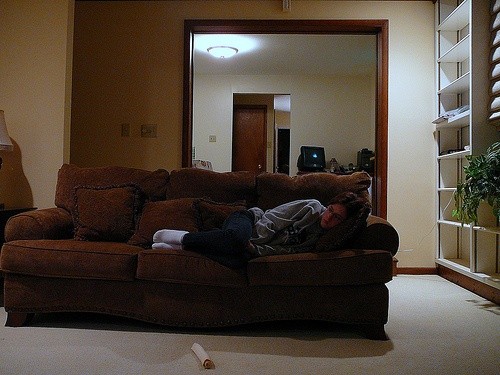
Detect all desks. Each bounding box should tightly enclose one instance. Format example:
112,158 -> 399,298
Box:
0,206 -> 37,308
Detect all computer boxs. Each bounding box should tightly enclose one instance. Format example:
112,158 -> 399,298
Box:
357,150 -> 375,177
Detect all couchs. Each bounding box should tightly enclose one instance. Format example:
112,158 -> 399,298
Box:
1,164 -> 399,339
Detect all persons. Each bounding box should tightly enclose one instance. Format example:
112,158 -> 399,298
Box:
152,192 -> 362,271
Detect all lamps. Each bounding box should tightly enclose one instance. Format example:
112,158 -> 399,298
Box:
207,47 -> 238,58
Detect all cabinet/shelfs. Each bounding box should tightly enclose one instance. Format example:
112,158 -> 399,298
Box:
433,0 -> 500,306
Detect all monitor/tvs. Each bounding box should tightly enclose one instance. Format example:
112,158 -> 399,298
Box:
297,146 -> 325,172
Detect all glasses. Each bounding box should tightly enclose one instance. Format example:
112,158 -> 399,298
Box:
326,206 -> 342,221
